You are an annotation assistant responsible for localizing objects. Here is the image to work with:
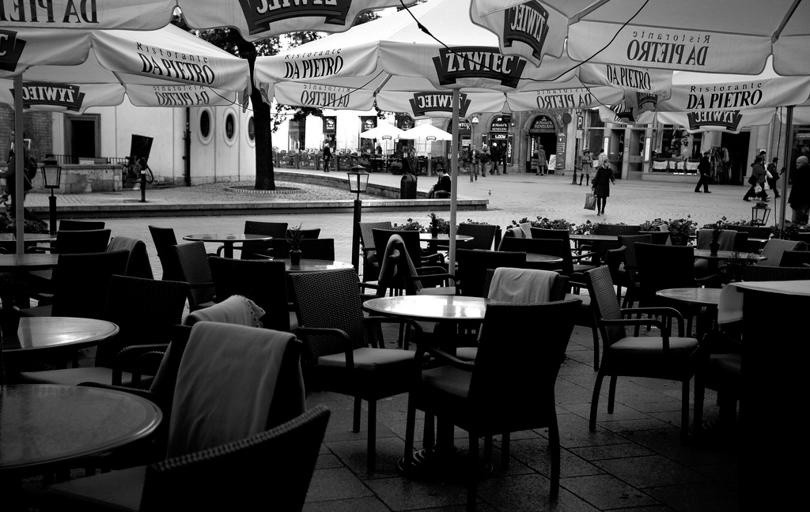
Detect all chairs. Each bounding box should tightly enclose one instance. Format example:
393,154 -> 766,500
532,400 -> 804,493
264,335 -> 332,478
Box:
18,277 -> 191,384
584,265 -> 700,436
286,267 -> 435,481
135,404 -> 333,511
76,294 -> 266,456
423,265 -> 571,470
272,146 -> 452,179
0,215 -> 808,334
704,281 -> 809,462
40,321 -> 304,510
422,295 -> 583,510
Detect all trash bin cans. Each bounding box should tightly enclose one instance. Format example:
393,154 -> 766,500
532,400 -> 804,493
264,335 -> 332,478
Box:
400,175 -> 417,199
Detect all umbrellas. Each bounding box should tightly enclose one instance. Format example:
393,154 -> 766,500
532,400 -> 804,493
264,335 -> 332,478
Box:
399,124 -> 452,176
0,0 -> 429,42
253,1 -> 673,449
360,123 -> 405,173
468,1 -> 810,77
599,55 -> 810,230
0,25 -> 252,253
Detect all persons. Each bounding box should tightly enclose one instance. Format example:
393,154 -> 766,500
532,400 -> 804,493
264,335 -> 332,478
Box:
577,149 -> 592,185
591,157 -> 616,216
743,149 -> 809,229
694,152 -> 712,193
536,145 -> 546,176
597,148 -> 608,169
0,128 -> 38,233
322,142 -> 333,172
467,137 -> 511,182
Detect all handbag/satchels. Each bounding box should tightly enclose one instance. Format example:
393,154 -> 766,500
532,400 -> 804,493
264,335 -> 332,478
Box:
755,184 -> 762,193
584,192 -> 596,209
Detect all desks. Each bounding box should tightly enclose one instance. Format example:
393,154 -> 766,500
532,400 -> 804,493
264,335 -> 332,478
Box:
653,287 -> 768,491
0,315 -> 119,383
2,381 -> 162,511
362,294 -> 496,482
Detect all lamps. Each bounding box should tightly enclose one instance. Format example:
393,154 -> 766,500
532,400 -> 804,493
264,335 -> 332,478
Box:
750,202 -> 771,227
39,152 -> 62,236
347,167 -> 370,275
